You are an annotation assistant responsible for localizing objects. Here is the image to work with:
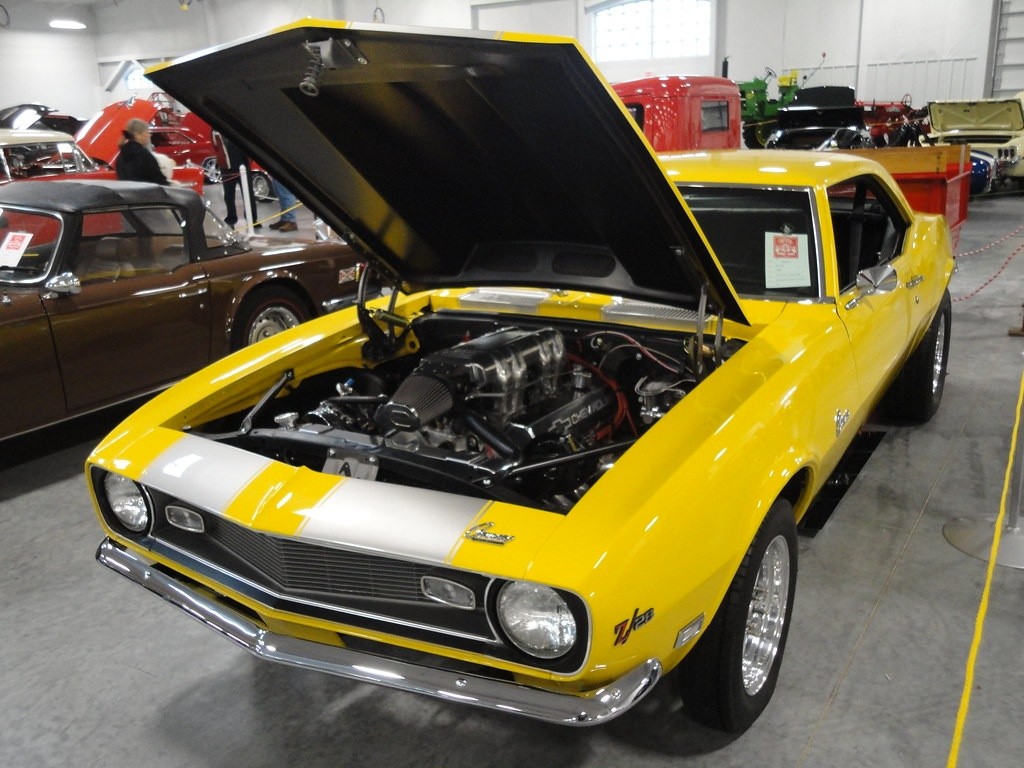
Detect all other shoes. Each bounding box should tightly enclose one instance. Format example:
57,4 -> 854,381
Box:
253,221 -> 262,228
269,220 -> 298,232
227,223 -> 234,230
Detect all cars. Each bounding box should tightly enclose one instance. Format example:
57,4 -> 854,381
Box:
147,111 -> 227,184
247,158 -> 277,202
1,103 -> 90,166
86,15 -> 962,750
914,88 -> 1024,188
0,177 -> 379,446
765,129 -> 997,197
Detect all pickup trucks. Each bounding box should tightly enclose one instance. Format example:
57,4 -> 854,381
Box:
609,75 -> 971,238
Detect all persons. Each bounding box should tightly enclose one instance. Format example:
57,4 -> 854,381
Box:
116,118 -> 173,230
210,128 -> 263,228
269,176 -> 297,231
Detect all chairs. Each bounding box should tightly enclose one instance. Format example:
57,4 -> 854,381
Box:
160,245 -> 185,268
74,237 -> 135,277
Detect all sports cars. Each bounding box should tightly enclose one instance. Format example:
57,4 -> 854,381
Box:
0,97 -> 204,250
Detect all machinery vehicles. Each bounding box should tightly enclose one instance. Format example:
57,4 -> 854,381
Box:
721,55 -> 803,148
